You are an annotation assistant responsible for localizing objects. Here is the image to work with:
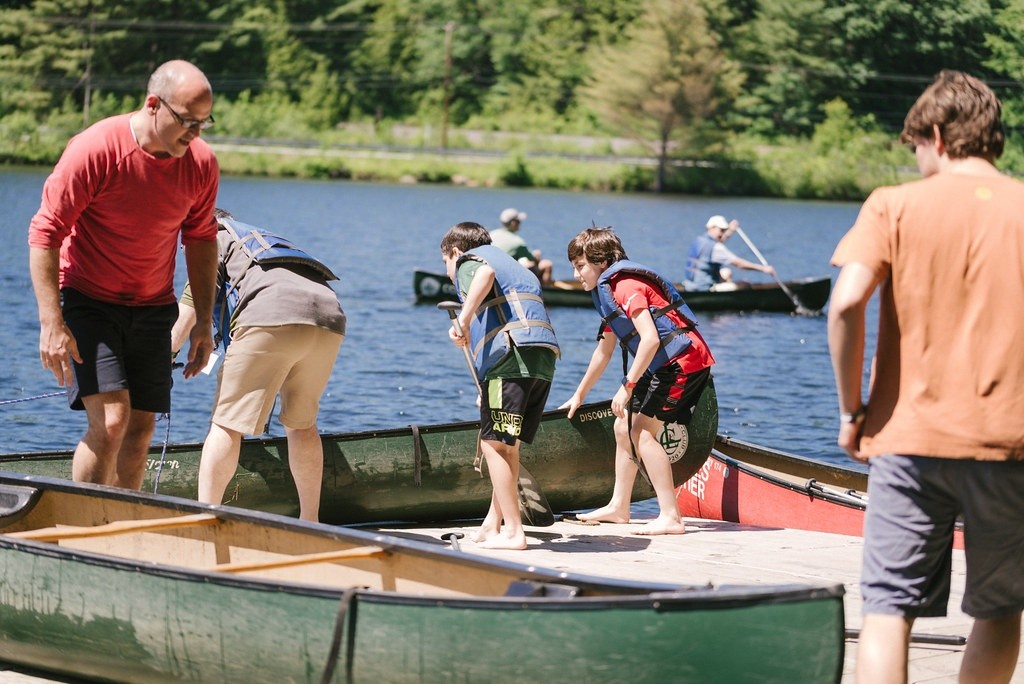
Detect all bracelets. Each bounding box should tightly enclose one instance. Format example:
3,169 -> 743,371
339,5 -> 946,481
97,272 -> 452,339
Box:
171,350 -> 181,358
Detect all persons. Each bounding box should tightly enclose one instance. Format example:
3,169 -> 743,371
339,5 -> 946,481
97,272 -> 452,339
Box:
488,209 -> 552,282
28,59 -> 219,492
558,226 -> 716,535
827,69 -> 1024,684
440,222 -> 561,551
684,216 -> 775,291
170,208 -> 346,523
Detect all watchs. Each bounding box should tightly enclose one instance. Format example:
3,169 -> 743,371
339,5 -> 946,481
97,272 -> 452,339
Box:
841,406 -> 866,422
621,376 -> 637,388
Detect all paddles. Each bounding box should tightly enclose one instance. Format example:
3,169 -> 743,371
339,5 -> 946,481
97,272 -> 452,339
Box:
735,224 -> 823,317
844,488 -> 869,501
440,532 -> 465,555
805,477 -> 869,508
438,300 -> 555,527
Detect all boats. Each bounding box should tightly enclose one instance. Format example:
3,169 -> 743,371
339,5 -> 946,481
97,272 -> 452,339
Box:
671,430 -> 966,551
0,470 -> 846,683
0,373 -> 719,523
410,267 -> 835,316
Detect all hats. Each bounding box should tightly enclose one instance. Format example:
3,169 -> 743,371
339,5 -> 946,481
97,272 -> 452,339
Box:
706,216 -> 729,230
500,208 -> 527,223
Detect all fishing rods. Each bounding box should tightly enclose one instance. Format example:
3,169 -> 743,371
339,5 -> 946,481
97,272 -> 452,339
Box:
0,363 -> 185,405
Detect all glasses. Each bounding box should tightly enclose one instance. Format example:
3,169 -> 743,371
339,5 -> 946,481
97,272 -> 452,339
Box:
910,142 -> 919,153
157,96 -> 215,130
